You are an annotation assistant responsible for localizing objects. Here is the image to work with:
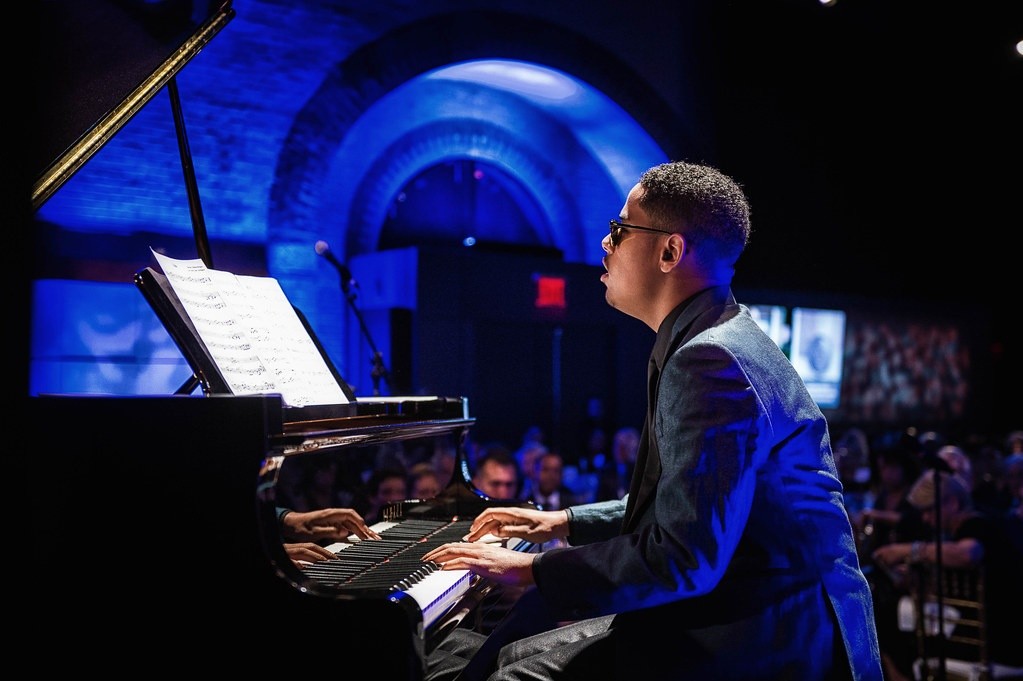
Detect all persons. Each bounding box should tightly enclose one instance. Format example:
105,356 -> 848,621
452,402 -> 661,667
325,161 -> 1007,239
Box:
420,160 -> 888,681
829,422 -> 1023,638
847,323 -> 966,409
254,466 -> 382,575
371,424 -> 641,513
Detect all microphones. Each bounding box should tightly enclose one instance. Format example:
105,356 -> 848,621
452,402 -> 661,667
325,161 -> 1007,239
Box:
919,432 -> 940,474
314,240 -> 359,289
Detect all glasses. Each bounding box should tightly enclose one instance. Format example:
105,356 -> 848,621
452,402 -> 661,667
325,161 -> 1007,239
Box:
607,218 -> 680,247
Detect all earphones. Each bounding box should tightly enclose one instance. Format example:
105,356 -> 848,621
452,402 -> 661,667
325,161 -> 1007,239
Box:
663,250 -> 673,259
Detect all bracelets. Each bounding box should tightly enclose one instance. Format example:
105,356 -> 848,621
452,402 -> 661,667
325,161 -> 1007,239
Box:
911,540 -> 928,562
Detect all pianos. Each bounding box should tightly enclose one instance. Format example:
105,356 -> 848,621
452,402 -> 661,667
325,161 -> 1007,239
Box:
0,0 -> 546,680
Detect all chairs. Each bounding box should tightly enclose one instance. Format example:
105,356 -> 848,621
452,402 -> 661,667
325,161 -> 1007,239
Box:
911,561 -> 1023,681
